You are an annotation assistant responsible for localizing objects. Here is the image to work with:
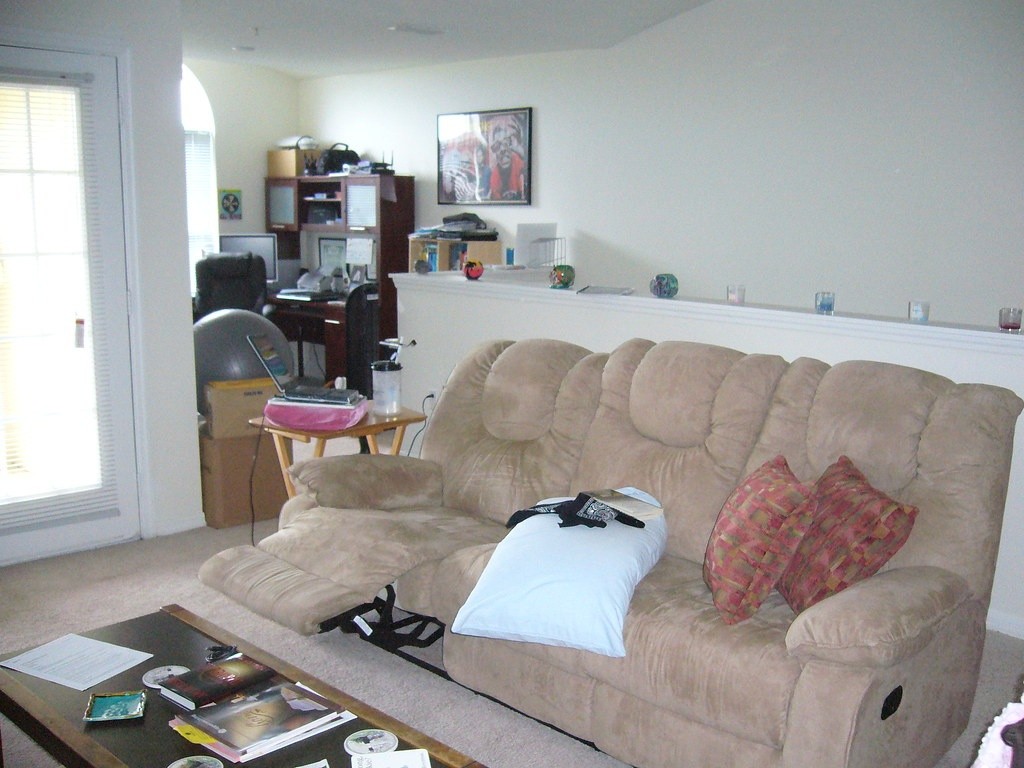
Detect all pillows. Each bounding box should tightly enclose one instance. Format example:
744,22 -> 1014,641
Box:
450,485 -> 667,658
702,453 -> 819,626
777,456 -> 920,616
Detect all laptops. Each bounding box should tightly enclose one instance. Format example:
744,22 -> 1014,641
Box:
247,333 -> 358,404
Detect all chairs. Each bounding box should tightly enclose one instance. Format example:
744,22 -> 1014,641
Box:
192,249 -> 267,322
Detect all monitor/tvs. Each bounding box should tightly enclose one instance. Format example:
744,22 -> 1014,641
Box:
217,233 -> 278,284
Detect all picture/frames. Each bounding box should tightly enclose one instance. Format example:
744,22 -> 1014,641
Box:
436,107 -> 534,205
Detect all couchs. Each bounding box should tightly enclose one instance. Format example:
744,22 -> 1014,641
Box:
198,336 -> 1024,768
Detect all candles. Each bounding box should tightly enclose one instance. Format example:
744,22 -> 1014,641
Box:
814,292 -> 835,314
908,300 -> 931,323
999,307 -> 1022,332
727,284 -> 746,305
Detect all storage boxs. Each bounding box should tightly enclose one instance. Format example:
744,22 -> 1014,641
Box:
199,426 -> 295,528
267,150 -> 323,178
202,377 -> 285,439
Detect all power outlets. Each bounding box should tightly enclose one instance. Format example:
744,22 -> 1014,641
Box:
426,388 -> 438,411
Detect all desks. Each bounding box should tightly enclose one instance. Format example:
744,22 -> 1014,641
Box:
250,400 -> 428,500
264,174 -> 415,399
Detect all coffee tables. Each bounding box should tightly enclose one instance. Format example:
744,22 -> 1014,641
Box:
0,604 -> 490,768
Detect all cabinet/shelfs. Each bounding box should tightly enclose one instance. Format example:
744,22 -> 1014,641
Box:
408,238 -> 503,273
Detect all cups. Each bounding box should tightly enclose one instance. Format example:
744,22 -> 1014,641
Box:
909,299 -> 930,322
333,275 -> 343,293
999,308 -> 1022,331
815,292 -> 835,315
727,284 -> 745,304
371,361 -> 403,416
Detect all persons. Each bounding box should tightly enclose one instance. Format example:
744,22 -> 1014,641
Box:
490,115 -> 524,199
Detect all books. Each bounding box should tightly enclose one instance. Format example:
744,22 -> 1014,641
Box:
159,652 -> 279,710
169,682 -> 357,763
